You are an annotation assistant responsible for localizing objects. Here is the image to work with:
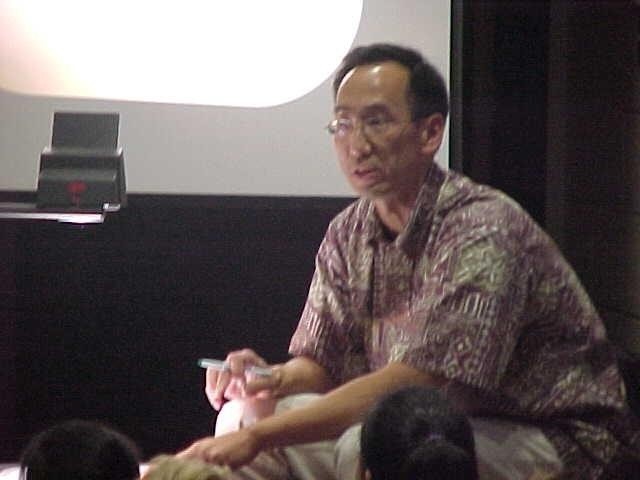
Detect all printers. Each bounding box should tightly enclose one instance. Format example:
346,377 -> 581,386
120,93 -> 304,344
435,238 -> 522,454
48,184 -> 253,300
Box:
35,110 -> 128,213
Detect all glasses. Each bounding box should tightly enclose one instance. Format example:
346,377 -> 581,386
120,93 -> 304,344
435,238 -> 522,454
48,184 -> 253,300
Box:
327,116 -> 393,134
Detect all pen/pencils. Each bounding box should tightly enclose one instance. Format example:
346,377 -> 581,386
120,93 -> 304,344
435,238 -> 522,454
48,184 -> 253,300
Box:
196,358 -> 273,376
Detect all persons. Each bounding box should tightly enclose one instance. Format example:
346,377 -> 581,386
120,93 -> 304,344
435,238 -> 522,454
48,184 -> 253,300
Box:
21,420 -> 140,480
175,45 -> 640,480
360,387 -> 478,480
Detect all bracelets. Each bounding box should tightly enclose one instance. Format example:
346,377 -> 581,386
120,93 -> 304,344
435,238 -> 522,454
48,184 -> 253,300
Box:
269,364 -> 292,399
